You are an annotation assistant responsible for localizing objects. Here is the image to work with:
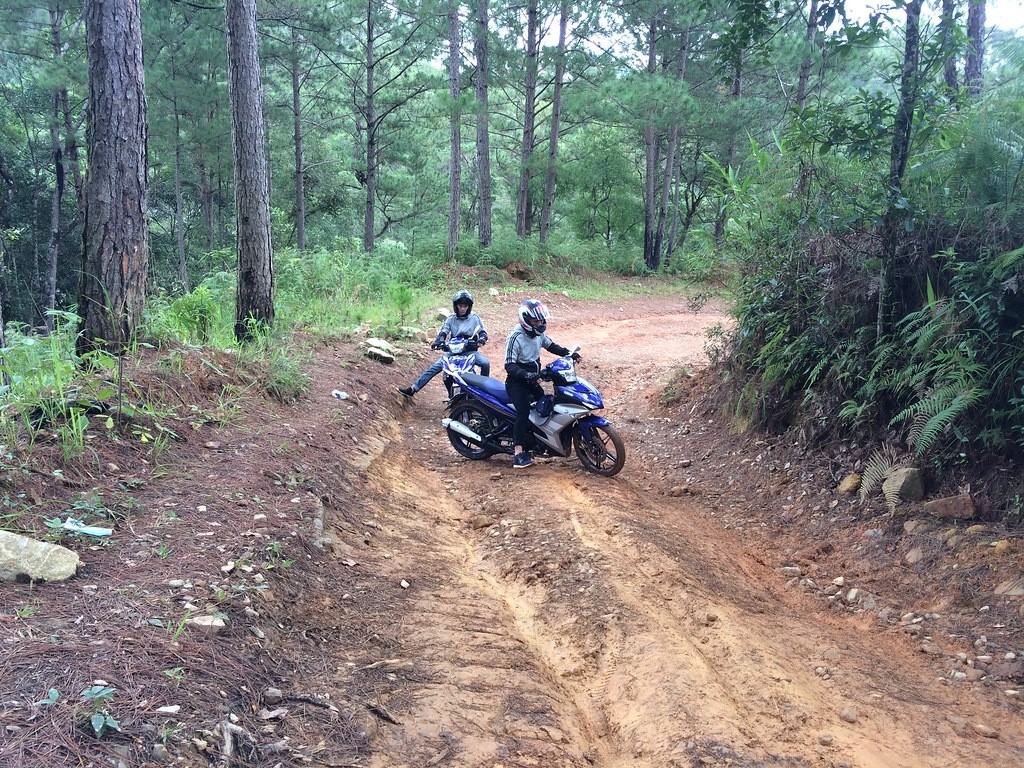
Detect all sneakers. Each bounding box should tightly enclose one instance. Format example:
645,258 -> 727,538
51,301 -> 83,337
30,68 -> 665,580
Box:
396,386 -> 413,399
512,452 -> 534,468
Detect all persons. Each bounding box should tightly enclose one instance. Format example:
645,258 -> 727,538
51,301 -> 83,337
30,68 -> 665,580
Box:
504,300 -> 583,468
399,290 -> 490,397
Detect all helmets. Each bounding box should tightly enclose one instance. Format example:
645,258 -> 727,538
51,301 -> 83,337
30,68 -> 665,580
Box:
453,290 -> 473,314
518,299 -> 550,338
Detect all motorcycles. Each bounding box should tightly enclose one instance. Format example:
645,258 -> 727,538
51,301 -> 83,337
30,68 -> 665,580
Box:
430,324 -> 484,397
442,344 -> 626,477
